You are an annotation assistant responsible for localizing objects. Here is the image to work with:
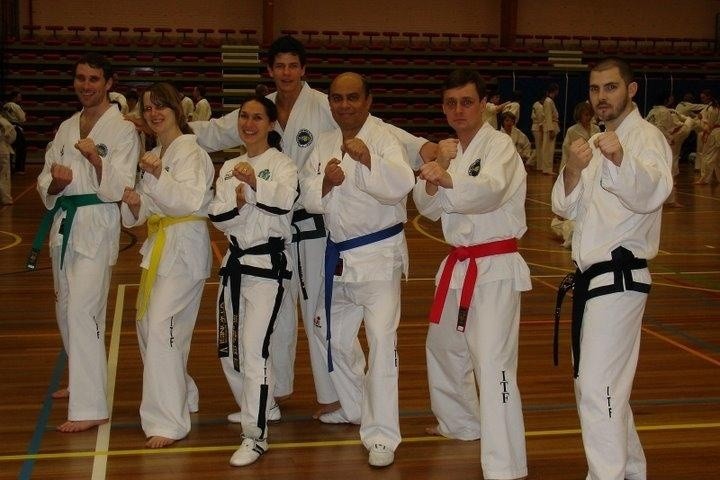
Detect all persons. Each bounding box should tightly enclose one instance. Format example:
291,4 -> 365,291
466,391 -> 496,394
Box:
209,96 -> 301,467
0,115 -> 17,204
122,82 -> 216,447
483,87 -> 720,248
126,37 -> 442,420
551,55 -> 675,480
1,90 -> 27,175
298,71 -> 414,467
412,66 -> 533,480
36,55 -> 139,433
108,71 -> 213,177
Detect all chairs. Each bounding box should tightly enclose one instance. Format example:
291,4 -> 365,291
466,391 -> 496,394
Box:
0,23 -> 720,152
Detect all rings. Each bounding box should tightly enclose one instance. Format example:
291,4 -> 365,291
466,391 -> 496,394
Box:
243,168 -> 248,173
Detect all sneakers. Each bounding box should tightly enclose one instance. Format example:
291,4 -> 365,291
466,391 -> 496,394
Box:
228,403 -> 282,424
321,410 -> 347,425
229,438 -> 268,467
369,445 -> 395,467
